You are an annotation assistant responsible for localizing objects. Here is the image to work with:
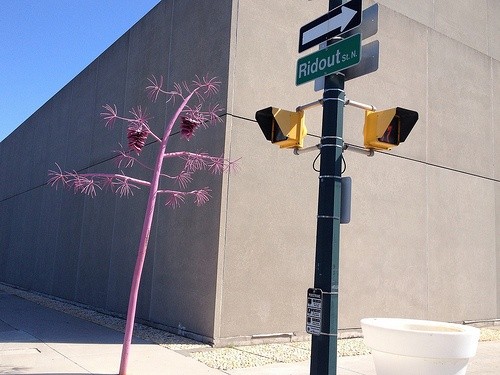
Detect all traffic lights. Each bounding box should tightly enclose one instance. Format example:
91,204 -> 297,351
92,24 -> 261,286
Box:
254,106 -> 307,150
362,106 -> 421,151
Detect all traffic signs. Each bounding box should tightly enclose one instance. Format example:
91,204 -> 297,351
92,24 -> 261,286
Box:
296,34 -> 361,86
